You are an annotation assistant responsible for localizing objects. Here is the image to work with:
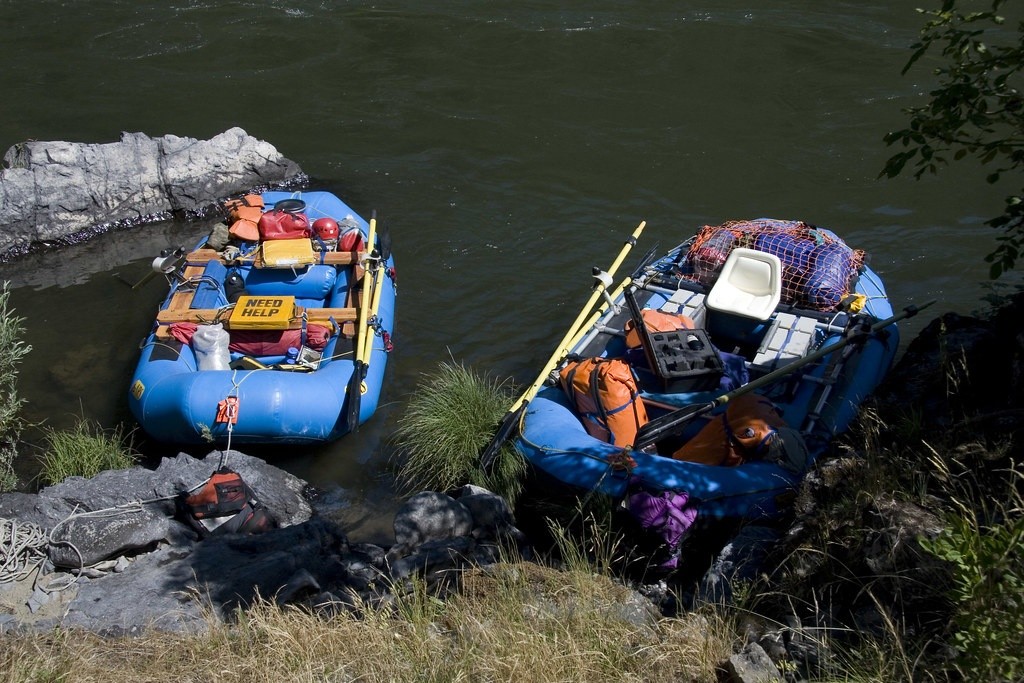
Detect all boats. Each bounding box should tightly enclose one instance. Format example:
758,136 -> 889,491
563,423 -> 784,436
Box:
514,220 -> 904,525
127,186 -> 400,454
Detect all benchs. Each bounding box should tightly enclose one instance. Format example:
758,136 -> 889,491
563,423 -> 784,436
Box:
186,252 -> 363,265
157,308 -> 359,325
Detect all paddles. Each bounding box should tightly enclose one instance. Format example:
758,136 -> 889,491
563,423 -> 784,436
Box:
346,207 -> 392,436
478,219 -> 662,474
632,296 -> 940,454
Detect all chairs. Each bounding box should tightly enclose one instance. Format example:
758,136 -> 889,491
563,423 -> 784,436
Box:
704,247 -> 782,321
